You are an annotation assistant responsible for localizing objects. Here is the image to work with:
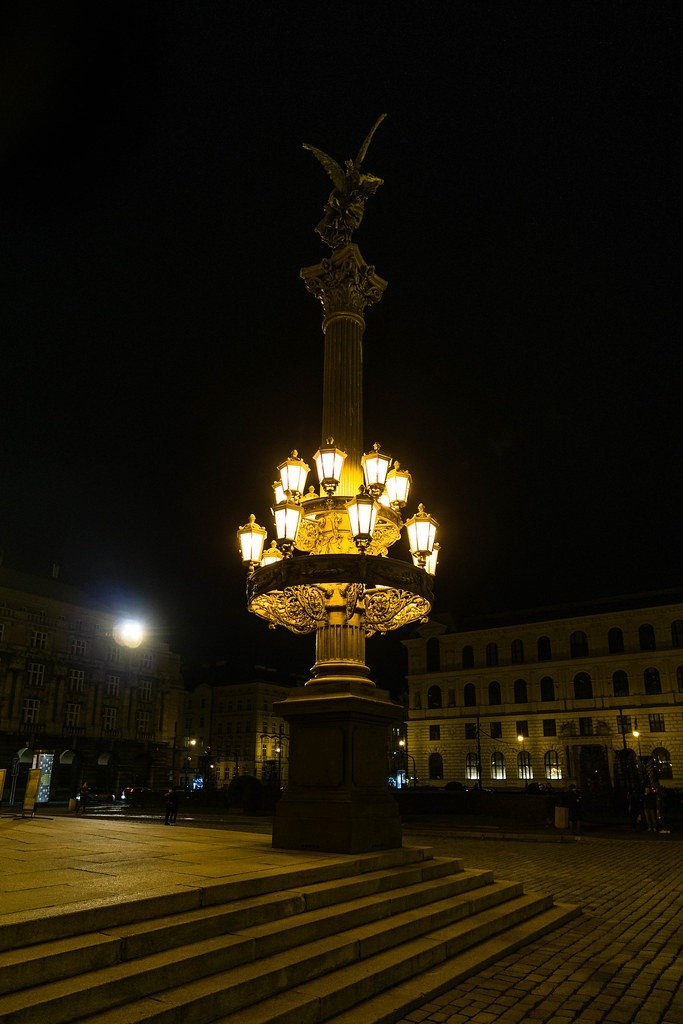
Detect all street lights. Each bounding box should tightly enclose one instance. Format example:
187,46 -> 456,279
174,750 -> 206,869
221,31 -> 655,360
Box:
633,730 -> 642,766
275,748 -> 281,783
517,735 -> 528,788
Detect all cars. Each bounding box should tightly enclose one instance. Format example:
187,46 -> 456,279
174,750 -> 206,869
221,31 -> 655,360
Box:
76,789 -> 115,804
121,786 -> 155,803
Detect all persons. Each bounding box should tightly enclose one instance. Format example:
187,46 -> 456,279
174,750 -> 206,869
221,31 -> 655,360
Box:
640,787 -> 658,832
76,789 -> 87,814
565,786 -> 583,821
164,788 -> 178,824
625,787 -> 642,832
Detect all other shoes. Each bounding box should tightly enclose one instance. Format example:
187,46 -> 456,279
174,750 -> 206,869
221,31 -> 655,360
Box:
648,828 -> 651,831
165,822 -> 170,825
171,823 -> 176,825
653,828 -> 657,832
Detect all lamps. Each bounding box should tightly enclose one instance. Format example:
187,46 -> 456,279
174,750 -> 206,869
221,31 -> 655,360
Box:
232,436 -> 442,578
